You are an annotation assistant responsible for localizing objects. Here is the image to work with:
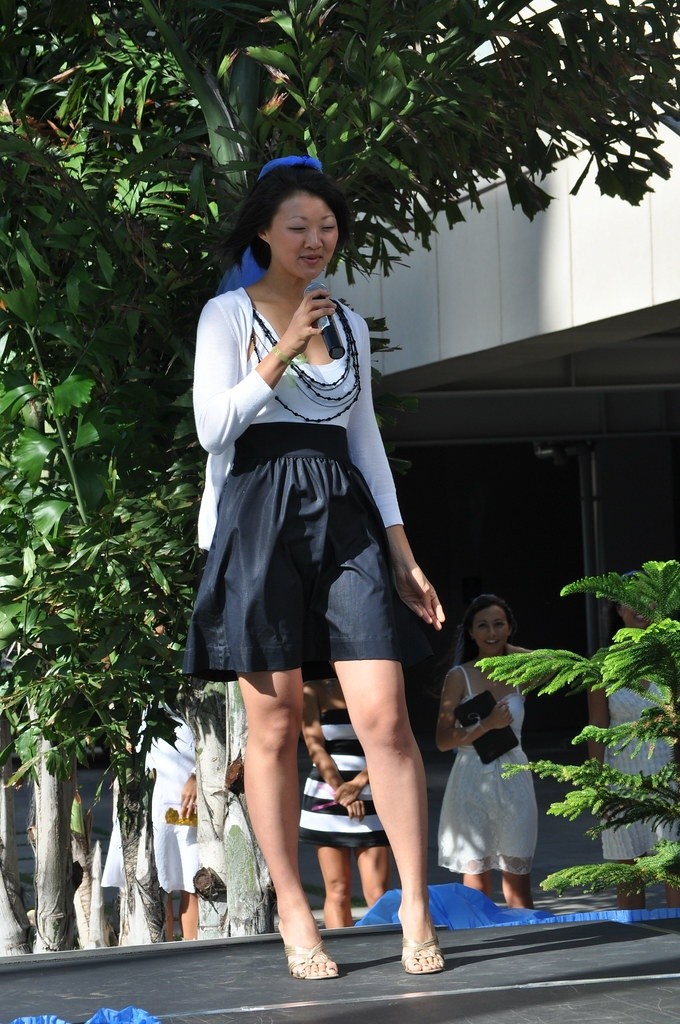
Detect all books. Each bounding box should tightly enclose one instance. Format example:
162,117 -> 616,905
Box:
454,690 -> 519,764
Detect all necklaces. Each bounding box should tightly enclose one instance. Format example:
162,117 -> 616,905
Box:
251,299 -> 361,422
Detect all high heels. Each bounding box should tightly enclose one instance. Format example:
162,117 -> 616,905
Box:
285,940 -> 340,979
401,936 -> 445,974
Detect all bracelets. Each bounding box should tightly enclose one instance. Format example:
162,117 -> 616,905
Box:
462,713 -> 486,743
191,768 -> 196,775
272,347 -> 291,364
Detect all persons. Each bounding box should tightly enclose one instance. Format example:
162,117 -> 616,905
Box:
181,157 -> 445,980
587,571 -> 680,908
102,702 -> 197,940
437,595 -> 557,909
300,659 -> 390,930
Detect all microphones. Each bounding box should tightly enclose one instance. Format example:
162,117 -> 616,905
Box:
305,280 -> 345,360
